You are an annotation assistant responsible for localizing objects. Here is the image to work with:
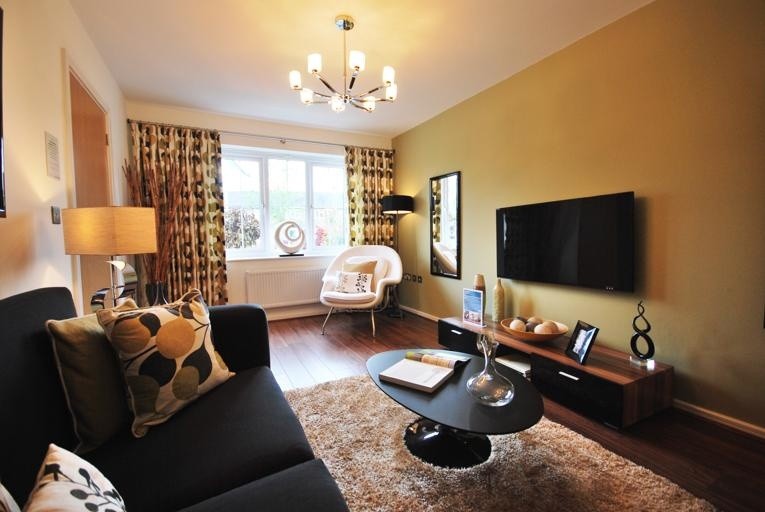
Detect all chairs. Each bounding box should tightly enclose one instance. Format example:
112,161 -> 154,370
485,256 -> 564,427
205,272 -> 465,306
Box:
433,240 -> 457,277
318,244 -> 403,340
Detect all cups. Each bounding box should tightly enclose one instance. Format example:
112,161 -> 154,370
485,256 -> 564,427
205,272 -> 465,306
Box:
477,328 -> 496,355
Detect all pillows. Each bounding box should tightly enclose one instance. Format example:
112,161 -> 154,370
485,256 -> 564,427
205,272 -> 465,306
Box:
341,257 -> 379,293
21,439 -> 129,512
42,295 -> 149,445
333,270 -> 374,295
345,253 -> 389,283
93,285 -> 243,441
0,481 -> 23,512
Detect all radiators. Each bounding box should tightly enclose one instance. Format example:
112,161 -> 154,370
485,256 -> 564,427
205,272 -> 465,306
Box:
242,264 -> 328,310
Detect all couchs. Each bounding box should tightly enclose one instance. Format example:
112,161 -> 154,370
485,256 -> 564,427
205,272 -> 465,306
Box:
0,284 -> 353,512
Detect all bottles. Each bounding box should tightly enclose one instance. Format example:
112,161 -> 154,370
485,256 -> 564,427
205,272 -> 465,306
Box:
474,273 -> 487,317
464,339 -> 515,407
492,278 -> 505,322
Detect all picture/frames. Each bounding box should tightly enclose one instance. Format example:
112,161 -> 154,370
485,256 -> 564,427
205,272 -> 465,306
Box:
561,316 -> 602,367
0,7 -> 8,218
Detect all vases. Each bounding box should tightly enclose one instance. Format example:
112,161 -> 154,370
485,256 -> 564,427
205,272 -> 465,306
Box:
491,278 -> 508,323
473,271 -> 486,321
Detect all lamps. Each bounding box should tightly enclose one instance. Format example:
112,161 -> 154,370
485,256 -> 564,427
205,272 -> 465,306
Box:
57,202 -> 160,312
285,12 -> 399,120
376,192 -> 418,320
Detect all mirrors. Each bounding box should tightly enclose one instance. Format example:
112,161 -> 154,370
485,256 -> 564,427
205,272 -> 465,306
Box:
428,171 -> 462,280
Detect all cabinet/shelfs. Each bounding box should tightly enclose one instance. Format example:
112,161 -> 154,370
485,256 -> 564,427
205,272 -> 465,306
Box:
433,309 -> 680,434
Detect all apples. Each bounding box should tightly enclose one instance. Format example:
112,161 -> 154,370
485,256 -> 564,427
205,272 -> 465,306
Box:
510,316 -> 558,335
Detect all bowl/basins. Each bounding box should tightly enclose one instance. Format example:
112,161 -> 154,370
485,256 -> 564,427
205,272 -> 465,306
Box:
501,317 -> 569,342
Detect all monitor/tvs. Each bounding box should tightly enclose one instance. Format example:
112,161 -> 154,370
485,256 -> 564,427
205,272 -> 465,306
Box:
496,191 -> 635,292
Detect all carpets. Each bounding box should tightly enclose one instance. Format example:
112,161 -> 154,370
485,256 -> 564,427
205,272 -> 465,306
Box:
281,373 -> 723,511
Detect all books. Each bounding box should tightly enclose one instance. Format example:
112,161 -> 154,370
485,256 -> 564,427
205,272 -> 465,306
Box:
463,288 -> 484,326
378,350 -> 472,392
